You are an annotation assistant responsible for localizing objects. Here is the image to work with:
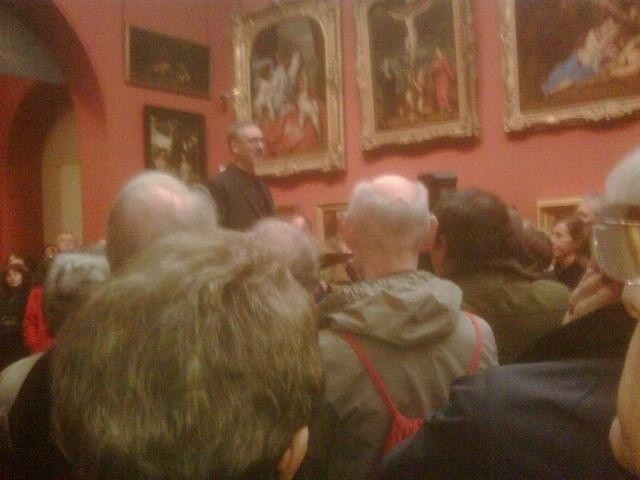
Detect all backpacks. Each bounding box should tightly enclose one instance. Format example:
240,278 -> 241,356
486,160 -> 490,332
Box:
339,309 -> 484,462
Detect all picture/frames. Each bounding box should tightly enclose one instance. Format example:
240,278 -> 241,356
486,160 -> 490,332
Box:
497,0 -> 640,134
144,105 -> 206,190
122,21 -> 212,100
310,198 -> 352,242
538,197 -> 586,232
355,0 -> 479,155
225,1 -> 345,178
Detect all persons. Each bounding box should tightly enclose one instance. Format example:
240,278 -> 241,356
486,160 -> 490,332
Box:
2,122 -> 639,476
